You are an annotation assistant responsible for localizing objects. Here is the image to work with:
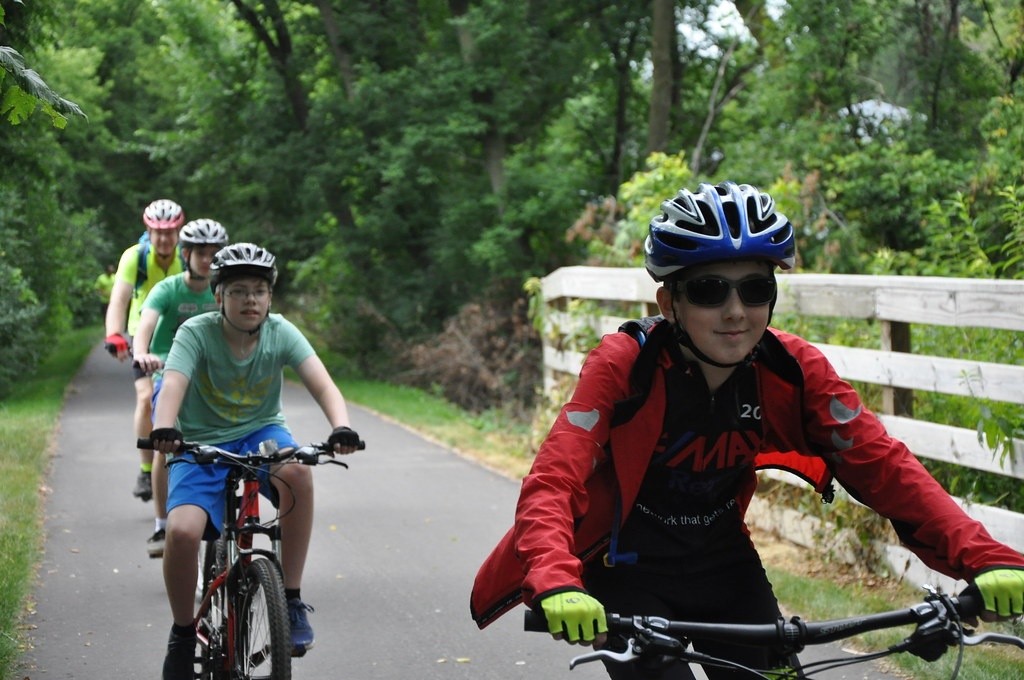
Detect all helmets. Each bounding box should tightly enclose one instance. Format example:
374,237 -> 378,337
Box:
178,218 -> 228,248
209,242 -> 277,294
143,199 -> 185,229
645,180 -> 796,283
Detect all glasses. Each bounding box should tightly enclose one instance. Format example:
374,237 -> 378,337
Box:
223,288 -> 271,300
664,274 -> 775,307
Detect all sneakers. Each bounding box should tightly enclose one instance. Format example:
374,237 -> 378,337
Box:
162,630 -> 195,680
288,596 -> 315,648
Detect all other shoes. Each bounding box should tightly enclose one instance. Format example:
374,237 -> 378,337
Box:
147,529 -> 166,554
133,472 -> 152,495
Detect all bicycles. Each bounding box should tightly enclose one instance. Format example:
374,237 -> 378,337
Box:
103,343 -> 174,503
524,584 -> 1023,680
132,360 -> 206,603
137,438 -> 366,680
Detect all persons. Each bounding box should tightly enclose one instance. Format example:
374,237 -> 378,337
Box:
95,262 -> 117,325
150,241 -> 361,680
132,218 -> 232,559
471,180 -> 1024,680
102,198 -> 187,503
